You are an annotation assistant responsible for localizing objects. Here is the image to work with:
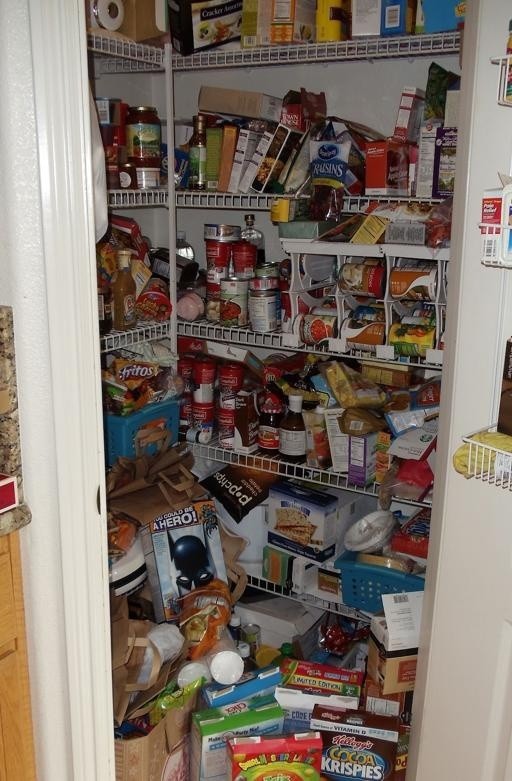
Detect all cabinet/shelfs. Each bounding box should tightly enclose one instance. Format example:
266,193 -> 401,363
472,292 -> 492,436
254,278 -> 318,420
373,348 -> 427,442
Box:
1,498 -> 37,780
86,33 -> 171,491
173,31 -> 465,627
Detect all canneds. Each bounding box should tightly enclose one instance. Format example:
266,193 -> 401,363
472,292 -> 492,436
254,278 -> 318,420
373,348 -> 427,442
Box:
293,258 -> 439,356
257,404 -> 283,455
125,106 -> 162,168
203,224 -> 279,333
241,623 -> 261,655
97,288 -> 112,335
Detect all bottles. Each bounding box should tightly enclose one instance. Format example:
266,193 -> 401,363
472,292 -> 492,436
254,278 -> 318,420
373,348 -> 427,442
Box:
228,614 -> 241,647
189,115 -> 207,192
241,214 -> 265,266
271,642 -> 298,665
176,231 -> 195,261
178,425 -> 209,445
279,394 -> 306,462
96,250 -> 136,334
238,642 -> 260,672
